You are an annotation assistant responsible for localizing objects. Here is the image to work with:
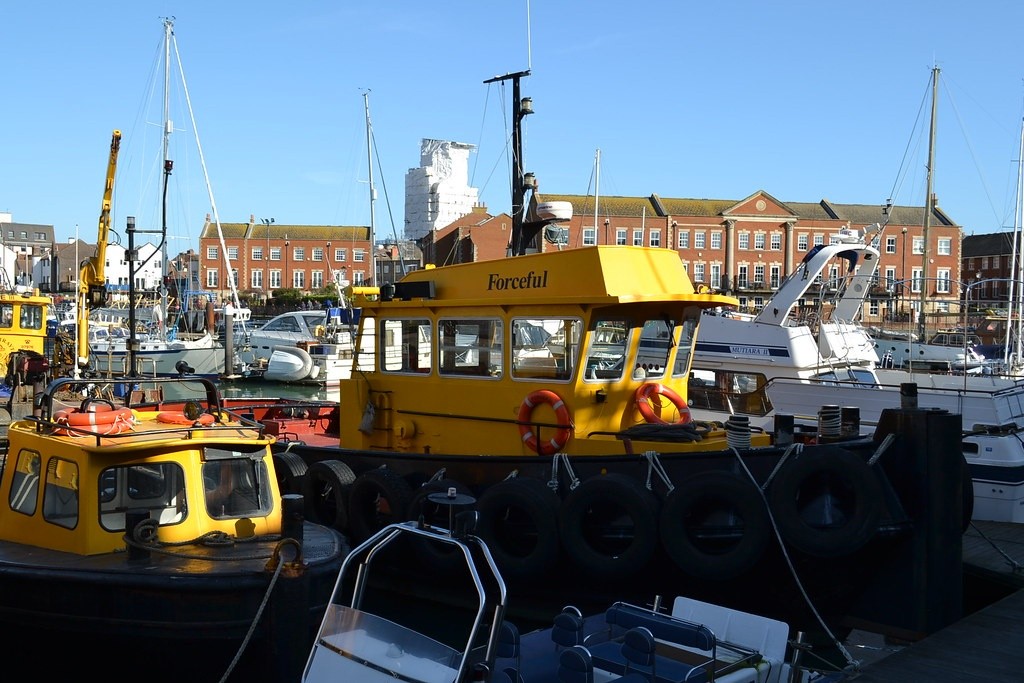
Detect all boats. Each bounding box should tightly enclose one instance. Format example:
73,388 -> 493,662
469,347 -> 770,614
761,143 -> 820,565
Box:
0,15 -> 1023,682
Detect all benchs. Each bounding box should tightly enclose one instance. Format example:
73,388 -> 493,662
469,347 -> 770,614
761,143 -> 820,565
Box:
584,601 -> 716,683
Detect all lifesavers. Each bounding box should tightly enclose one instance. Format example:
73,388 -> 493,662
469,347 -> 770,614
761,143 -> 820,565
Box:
314,324 -> 325,338
635,382 -> 691,429
51,404 -> 134,436
156,410 -> 215,424
517,388 -> 572,456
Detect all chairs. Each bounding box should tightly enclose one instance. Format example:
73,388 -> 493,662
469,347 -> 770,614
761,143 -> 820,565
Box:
551,605 -> 584,652
490,620 -> 521,683
620,626 -> 656,683
558,645 -> 595,683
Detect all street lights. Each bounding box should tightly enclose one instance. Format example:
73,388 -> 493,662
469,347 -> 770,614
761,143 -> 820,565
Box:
671,220 -> 678,251
285,241 -> 290,291
901,227 -> 908,316
975,269 -> 982,312
327,241 -> 332,281
260,218 -> 274,298
604,218 -> 610,245
